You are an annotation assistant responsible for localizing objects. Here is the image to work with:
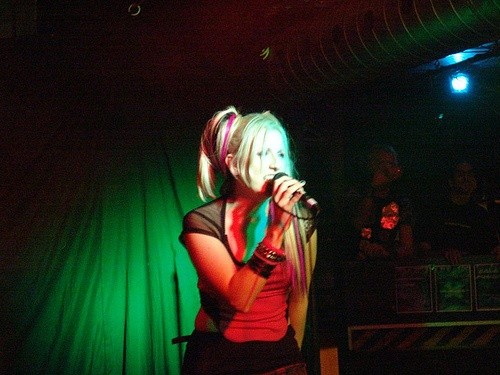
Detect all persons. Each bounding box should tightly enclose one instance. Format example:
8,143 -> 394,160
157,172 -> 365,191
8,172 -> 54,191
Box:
178,104 -> 312,375
352,144 -> 417,258
420,158 -> 500,266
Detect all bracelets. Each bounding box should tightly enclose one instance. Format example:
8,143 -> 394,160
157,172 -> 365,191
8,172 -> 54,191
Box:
249,238 -> 286,279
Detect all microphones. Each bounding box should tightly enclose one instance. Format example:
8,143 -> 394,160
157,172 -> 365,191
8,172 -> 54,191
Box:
273,172 -> 320,217
